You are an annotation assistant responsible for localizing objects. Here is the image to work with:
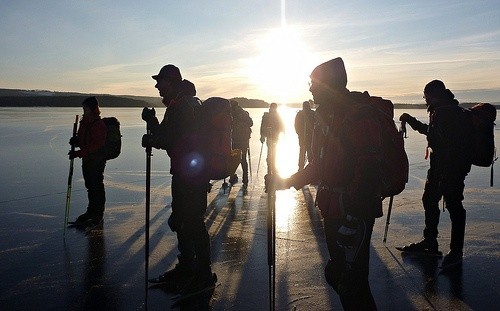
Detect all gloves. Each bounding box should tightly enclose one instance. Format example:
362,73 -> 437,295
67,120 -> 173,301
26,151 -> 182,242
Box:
68,150 -> 79,160
142,107 -> 155,122
142,133 -> 153,148
69,138 -> 79,147
260,137 -> 265,142
399,113 -> 417,128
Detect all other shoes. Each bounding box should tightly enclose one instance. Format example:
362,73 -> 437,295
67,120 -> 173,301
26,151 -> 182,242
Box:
84,217 -> 101,226
159,267 -> 182,282
402,236 -> 439,251
79,213 -> 90,221
443,249 -> 463,264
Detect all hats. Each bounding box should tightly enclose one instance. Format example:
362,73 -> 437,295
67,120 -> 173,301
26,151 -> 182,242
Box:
312,58 -> 347,90
425,80 -> 446,98
152,64 -> 181,81
82,95 -> 99,106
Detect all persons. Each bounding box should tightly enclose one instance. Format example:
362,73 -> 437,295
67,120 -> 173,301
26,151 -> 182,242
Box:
264,56 -> 383,311
260,103 -> 283,175
400,80 -> 472,260
228,100 -> 253,185
294,101 -> 317,171
65,96 -> 105,224
142,65 -> 212,292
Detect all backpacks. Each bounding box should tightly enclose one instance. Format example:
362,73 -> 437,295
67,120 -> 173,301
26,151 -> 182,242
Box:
87,117 -> 122,159
332,91 -> 409,198
171,97 -> 242,180
442,103 -> 497,167
263,114 -> 278,137
